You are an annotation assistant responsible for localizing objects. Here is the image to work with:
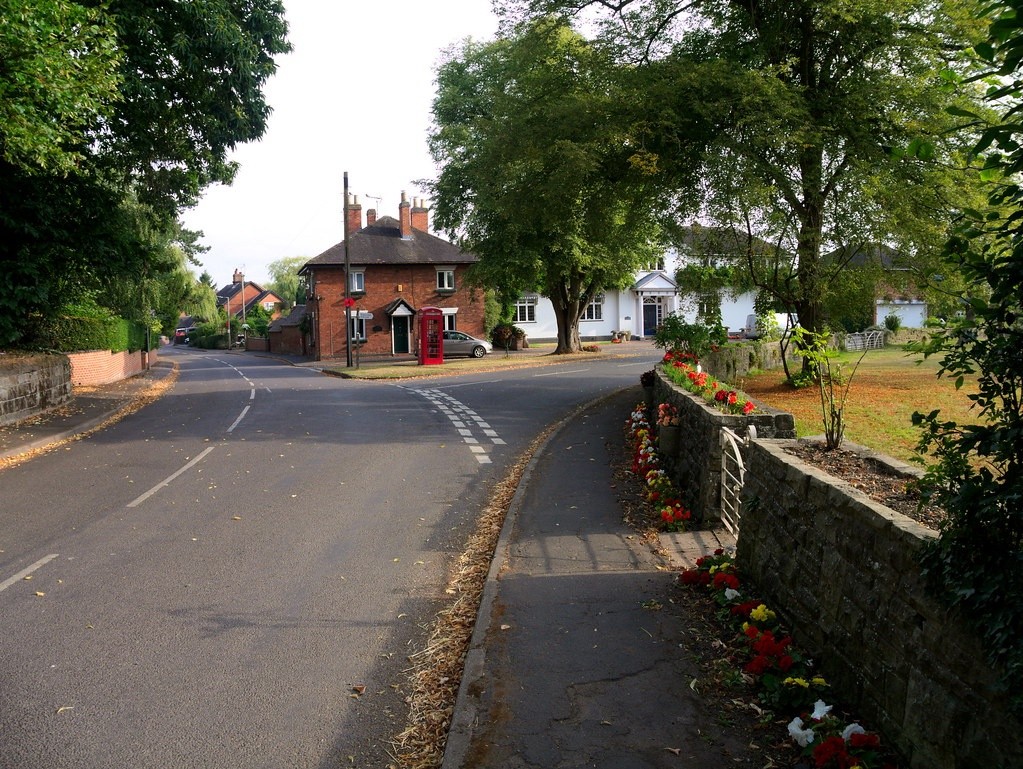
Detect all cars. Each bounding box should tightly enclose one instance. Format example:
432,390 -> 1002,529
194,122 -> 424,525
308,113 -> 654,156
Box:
414,330 -> 493,358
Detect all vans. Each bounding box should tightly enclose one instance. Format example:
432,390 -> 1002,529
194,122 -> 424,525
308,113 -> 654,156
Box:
746,313 -> 800,338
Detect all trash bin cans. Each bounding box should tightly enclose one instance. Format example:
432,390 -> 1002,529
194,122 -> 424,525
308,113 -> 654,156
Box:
176,336 -> 184,344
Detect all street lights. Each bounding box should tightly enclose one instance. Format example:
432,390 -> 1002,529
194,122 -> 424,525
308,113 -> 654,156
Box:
217,296 -> 231,350
